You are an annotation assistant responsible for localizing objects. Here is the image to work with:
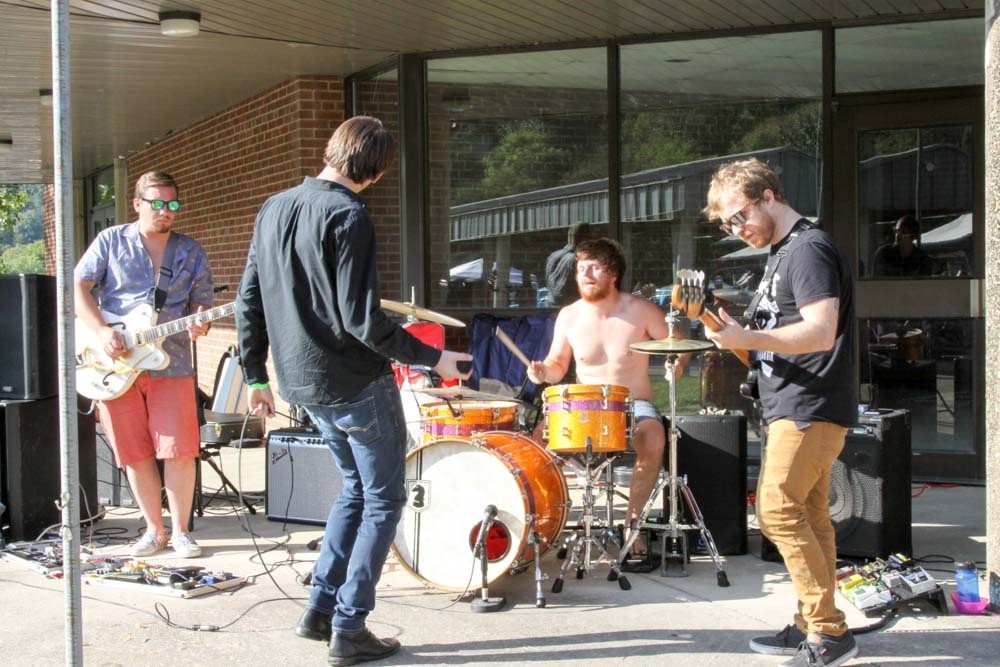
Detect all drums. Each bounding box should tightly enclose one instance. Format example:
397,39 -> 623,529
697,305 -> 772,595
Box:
392,431 -> 573,594
542,383 -> 634,454
418,399 -> 519,446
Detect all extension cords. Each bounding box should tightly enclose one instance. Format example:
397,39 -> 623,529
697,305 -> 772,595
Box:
175,576 -> 240,598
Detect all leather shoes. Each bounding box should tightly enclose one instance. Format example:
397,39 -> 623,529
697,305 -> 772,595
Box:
295,608 -> 334,640
328,626 -> 401,666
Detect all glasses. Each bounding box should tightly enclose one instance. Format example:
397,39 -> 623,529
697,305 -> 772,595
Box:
139,198 -> 180,212
720,196 -> 765,235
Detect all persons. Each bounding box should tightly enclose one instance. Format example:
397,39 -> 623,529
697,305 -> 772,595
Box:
877,215 -> 937,360
543,220 -> 593,308
73,171 -> 213,560
526,238 -> 692,564
233,116 -> 473,666
702,157 -> 858,667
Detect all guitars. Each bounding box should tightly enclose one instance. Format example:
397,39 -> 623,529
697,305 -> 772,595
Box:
667,268 -> 764,438
74,300 -> 237,400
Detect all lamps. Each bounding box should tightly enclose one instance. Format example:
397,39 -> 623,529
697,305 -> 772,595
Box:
0,140 -> 14,155
159,13 -> 200,37
39,89 -> 52,106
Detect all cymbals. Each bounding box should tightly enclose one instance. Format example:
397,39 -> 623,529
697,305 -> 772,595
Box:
403,386 -> 524,404
626,337 -> 715,355
380,299 -> 467,328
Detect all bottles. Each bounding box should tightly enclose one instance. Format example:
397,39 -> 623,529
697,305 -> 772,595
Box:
954,560 -> 980,603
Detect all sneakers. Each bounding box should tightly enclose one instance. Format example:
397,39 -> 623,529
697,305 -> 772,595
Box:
750,623 -> 807,656
172,533 -> 201,558
129,532 -> 168,556
784,628 -> 860,667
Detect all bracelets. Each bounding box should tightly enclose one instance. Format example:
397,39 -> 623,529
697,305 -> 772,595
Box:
246,383 -> 269,391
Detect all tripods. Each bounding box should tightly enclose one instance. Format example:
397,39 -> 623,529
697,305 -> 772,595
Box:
555,352 -> 730,597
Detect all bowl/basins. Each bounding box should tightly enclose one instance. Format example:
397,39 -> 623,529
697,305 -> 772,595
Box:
950,592 -> 988,614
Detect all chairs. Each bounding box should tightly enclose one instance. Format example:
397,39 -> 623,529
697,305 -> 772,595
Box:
162,343 -> 268,517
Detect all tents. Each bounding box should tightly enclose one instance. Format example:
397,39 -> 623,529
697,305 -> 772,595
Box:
447,258 -> 538,309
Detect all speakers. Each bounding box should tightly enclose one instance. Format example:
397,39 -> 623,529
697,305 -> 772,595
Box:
662,413 -> 748,554
0,274 -> 59,400
760,407 -> 912,563
265,426 -> 345,526
0,395 -> 97,541
